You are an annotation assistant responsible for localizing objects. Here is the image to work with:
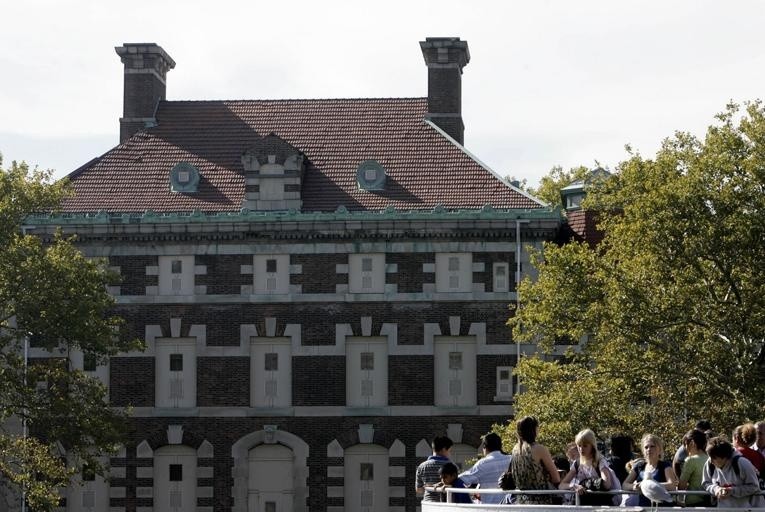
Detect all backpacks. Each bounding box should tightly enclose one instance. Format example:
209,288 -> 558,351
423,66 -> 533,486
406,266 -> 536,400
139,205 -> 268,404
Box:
574,459 -> 622,506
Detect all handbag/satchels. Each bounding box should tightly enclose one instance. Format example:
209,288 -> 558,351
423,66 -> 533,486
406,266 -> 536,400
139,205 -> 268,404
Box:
498,472 -> 516,490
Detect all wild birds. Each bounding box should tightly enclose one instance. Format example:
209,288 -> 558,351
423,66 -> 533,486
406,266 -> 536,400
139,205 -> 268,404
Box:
639,464 -> 674,512
